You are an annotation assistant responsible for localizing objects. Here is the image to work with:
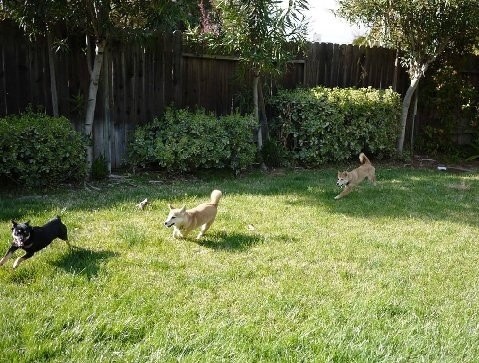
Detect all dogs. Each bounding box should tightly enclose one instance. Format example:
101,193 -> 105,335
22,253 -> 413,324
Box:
165,189 -> 222,240
334,153 -> 376,201
0,214 -> 71,269
